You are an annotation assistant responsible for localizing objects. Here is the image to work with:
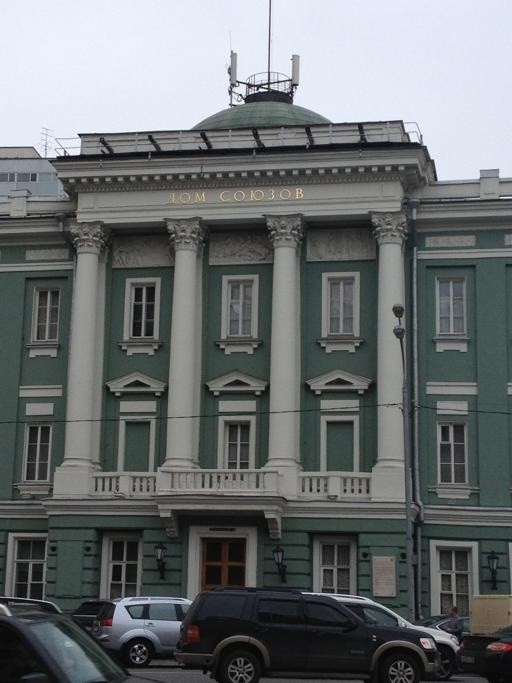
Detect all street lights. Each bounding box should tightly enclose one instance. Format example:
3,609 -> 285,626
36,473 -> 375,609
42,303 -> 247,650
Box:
392,303 -> 416,622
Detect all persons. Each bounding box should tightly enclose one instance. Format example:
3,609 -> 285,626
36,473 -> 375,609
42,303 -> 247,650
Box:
442,605 -> 465,640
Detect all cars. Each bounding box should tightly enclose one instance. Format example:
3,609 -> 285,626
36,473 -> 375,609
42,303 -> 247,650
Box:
0,596 -> 170,683
456,624 -> 512,683
410,614 -> 471,643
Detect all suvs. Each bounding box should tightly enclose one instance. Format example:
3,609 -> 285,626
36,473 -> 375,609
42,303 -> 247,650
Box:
172,585 -> 444,683
72,595 -> 193,668
301,591 -> 461,682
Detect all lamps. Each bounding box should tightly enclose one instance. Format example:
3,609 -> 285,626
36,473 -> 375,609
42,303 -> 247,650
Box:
153,540 -> 168,579
271,543 -> 288,584
487,550 -> 499,590
